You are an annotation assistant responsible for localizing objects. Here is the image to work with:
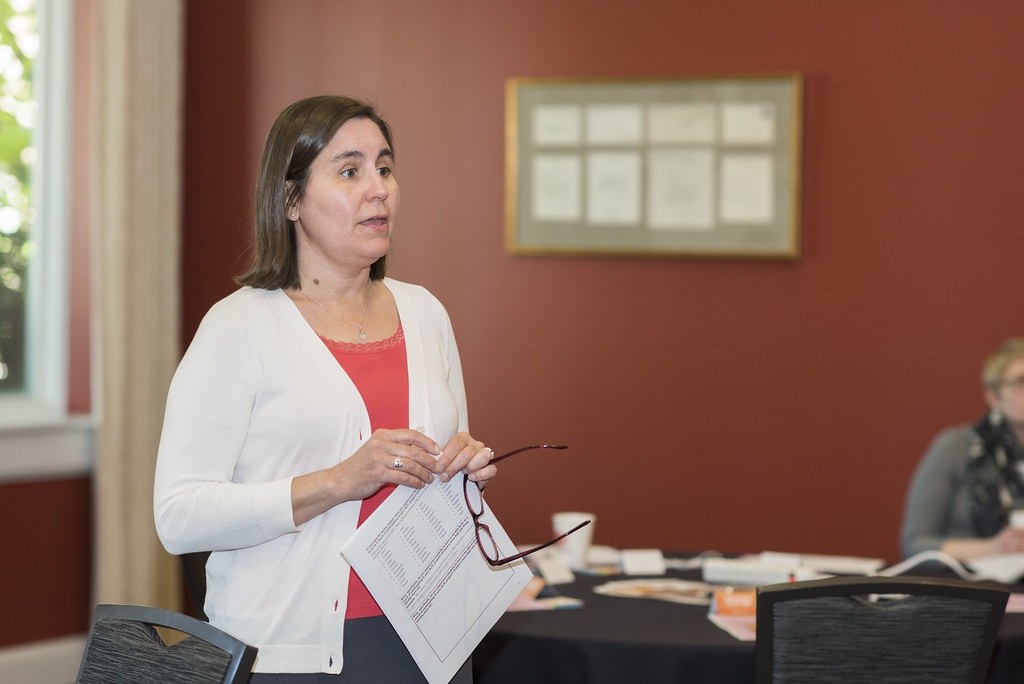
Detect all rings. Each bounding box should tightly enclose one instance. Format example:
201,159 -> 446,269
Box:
486,447 -> 494,458
394,457 -> 403,473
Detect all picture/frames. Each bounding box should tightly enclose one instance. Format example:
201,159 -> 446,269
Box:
500,70 -> 803,261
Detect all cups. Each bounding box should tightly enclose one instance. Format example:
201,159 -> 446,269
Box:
551,511 -> 598,570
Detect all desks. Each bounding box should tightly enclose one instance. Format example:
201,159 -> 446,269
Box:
469,553 -> 1024,684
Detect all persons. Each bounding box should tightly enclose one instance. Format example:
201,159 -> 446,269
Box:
903,339 -> 1024,590
153,97 -> 499,684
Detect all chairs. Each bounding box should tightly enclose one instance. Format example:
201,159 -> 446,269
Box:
755,574 -> 1010,684
76,604 -> 259,684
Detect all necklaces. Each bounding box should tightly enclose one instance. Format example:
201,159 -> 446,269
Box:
293,275 -> 371,340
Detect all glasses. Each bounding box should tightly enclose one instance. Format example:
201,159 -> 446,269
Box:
992,375 -> 1024,388
463,444 -> 591,567
